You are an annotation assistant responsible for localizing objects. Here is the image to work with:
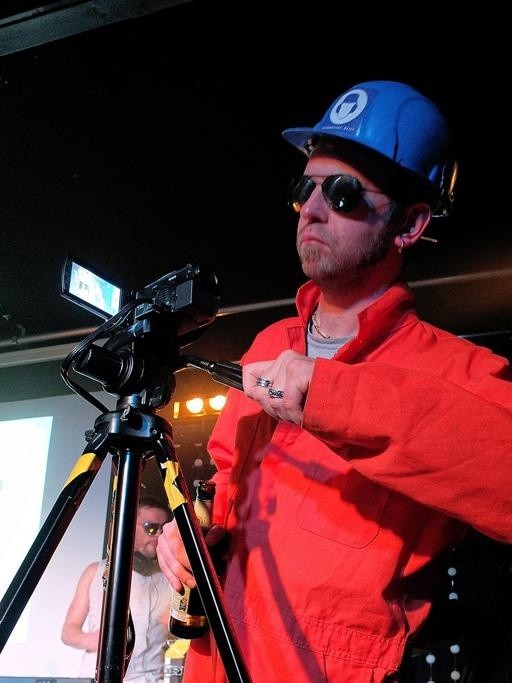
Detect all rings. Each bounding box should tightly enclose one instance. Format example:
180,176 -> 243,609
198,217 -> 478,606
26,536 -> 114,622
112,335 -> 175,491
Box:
256,375 -> 274,389
268,387 -> 284,398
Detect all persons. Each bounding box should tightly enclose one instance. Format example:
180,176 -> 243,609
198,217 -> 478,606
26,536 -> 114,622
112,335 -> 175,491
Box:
61,489 -> 171,682
150,78 -> 511,682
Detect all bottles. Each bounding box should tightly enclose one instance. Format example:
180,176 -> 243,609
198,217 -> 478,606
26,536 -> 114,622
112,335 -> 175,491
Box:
167,481 -> 217,640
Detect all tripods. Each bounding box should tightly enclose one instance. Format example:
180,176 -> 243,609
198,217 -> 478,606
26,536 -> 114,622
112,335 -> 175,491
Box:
0,395 -> 249,683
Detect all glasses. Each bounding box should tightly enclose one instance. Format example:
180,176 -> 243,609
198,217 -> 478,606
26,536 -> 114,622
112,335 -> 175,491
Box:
138,520 -> 163,537
285,171 -> 390,211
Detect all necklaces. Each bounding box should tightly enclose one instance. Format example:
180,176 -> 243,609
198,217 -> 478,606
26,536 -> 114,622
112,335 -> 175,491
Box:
311,311 -> 332,339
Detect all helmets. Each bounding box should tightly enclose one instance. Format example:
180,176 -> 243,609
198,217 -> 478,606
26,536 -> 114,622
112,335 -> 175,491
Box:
282,78 -> 458,201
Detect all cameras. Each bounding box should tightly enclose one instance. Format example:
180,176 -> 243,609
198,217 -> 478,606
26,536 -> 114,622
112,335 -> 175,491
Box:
60,253 -> 222,395
